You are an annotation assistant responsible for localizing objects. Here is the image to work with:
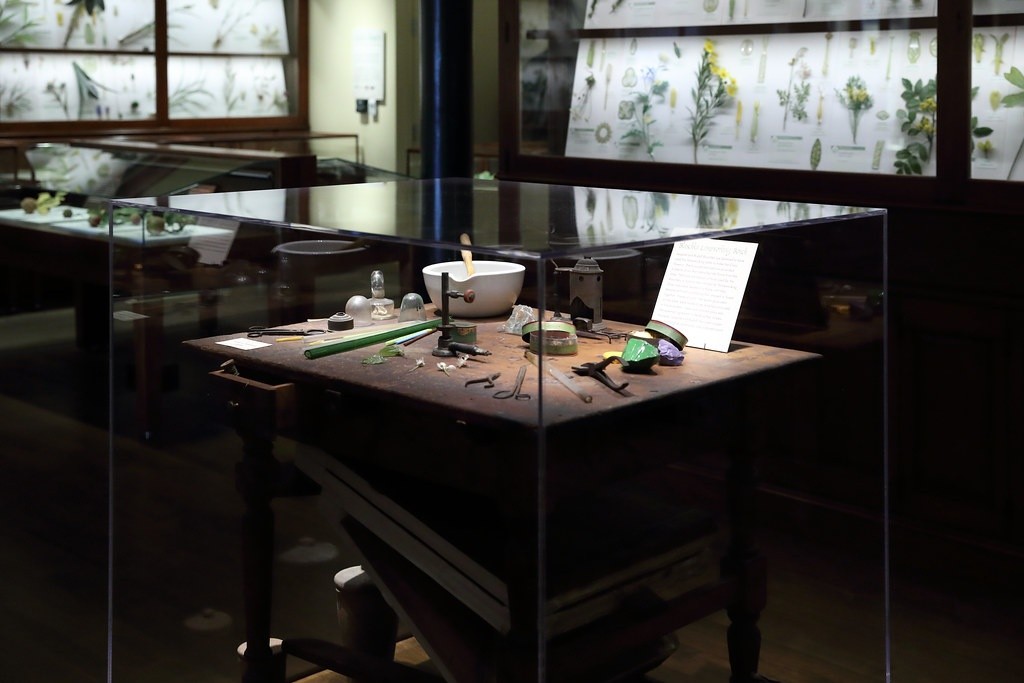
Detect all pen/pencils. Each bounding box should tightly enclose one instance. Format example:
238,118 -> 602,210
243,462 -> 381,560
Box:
385,327 -> 437,346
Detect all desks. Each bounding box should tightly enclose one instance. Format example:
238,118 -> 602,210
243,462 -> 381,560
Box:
0,204 -> 237,305
182,302 -> 824,683
535,383 -> 547,385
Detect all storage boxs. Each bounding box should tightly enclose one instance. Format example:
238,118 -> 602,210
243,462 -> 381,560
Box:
520,321 -> 578,355
626,319 -> 688,351
448,321 -> 477,343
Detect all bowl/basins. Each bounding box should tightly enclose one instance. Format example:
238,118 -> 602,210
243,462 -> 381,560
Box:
422,260 -> 525,317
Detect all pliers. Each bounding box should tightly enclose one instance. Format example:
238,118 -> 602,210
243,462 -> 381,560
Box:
575,354 -> 630,391
464,371 -> 501,388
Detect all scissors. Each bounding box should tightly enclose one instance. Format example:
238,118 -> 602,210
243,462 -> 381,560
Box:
491,365 -> 531,400
246,324 -> 327,337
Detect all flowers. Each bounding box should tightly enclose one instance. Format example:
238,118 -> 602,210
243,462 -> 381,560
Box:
0,0 -> 293,118
629,38 -> 999,177
575,186 -> 875,249
